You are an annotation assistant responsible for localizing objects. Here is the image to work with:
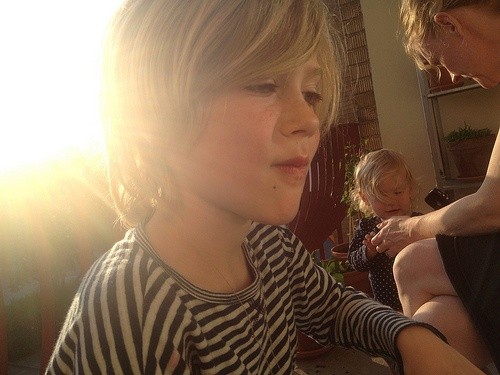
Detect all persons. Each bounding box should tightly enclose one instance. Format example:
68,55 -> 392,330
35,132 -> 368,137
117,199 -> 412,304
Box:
370,0 -> 500,371
346,147 -> 429,316
44,0 -> 489,375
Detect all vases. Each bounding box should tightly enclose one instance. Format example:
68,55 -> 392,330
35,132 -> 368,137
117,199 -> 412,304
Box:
424,59 -> 465,91
446,133 -> 495,181
298,329 -> 328,358
331,244 -> 348,260
344,269 -> 373,298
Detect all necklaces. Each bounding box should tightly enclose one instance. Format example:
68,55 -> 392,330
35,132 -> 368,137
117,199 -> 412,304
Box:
154,206 -> 284,375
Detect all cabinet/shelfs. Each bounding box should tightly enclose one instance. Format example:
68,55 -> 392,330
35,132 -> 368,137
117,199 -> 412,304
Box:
416,58 -> 485,197
